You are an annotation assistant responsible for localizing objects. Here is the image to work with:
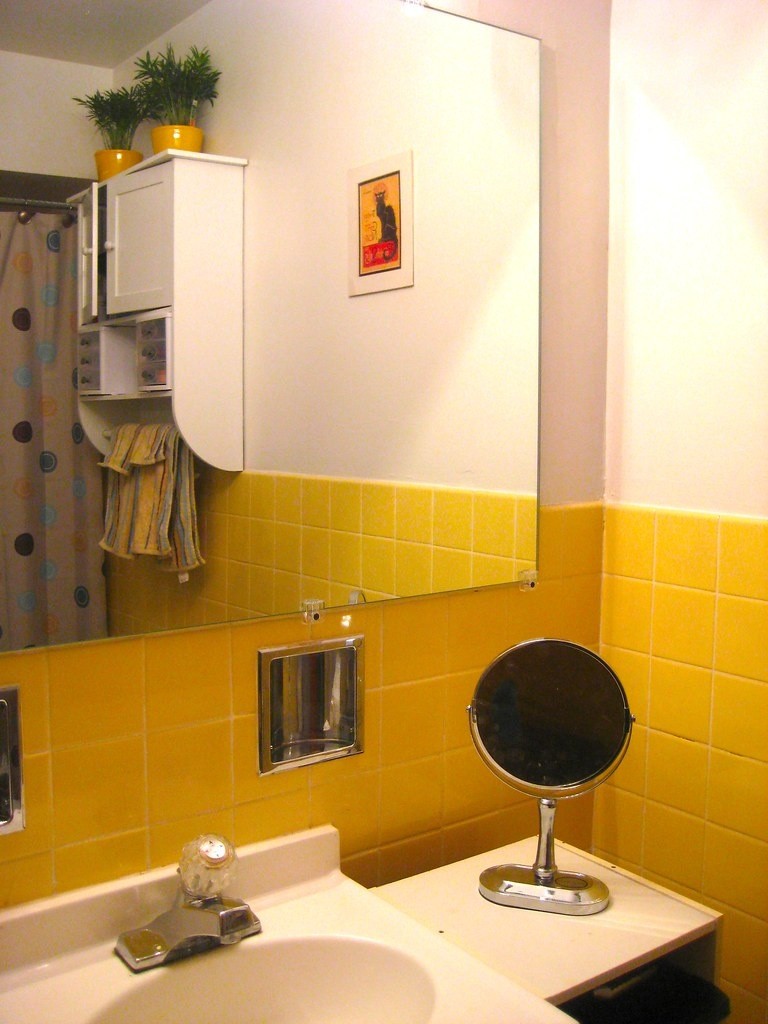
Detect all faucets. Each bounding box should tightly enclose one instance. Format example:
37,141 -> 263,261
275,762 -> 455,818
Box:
145,836 -> 255,950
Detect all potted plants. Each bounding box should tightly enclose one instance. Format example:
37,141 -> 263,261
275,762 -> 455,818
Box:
72,84 -> 149,183
133,41 -> 223,156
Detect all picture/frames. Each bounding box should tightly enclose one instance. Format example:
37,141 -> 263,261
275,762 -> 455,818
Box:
348,147 -> 415,298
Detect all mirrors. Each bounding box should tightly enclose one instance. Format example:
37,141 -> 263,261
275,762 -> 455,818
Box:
0,0 -> 542,649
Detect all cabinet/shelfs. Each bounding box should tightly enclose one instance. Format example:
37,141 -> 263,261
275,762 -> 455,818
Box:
66,150 -> 248,473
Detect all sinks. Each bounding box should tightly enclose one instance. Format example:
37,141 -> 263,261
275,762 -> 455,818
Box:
84,936 -> 439,1023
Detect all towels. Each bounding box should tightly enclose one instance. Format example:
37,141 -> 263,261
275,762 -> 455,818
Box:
96,422 -> 206,572
